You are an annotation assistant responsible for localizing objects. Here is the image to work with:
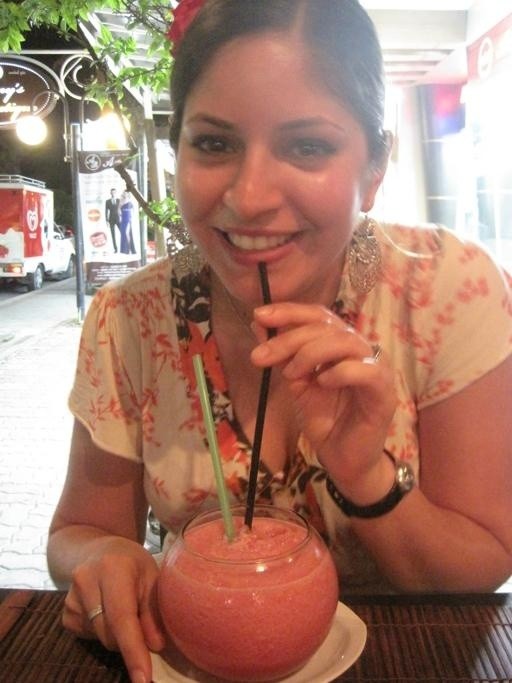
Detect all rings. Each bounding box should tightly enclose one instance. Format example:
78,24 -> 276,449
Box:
372,342 -> 384,360
86,605 -> 104,621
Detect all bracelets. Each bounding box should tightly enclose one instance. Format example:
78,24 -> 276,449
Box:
317,449 -> 414,517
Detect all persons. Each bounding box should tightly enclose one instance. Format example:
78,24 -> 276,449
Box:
116,190 -> 137,254
105,188 -> 123,252
45,0 -> 510,679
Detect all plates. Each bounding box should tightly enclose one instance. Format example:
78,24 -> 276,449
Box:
147,599 -> 369,683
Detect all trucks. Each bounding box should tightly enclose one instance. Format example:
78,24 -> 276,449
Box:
0,171 -> 75,292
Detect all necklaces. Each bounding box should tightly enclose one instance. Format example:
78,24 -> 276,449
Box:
221,286 -> 274,348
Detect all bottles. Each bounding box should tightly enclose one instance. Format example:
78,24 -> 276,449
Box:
157,502 -> 340,683
